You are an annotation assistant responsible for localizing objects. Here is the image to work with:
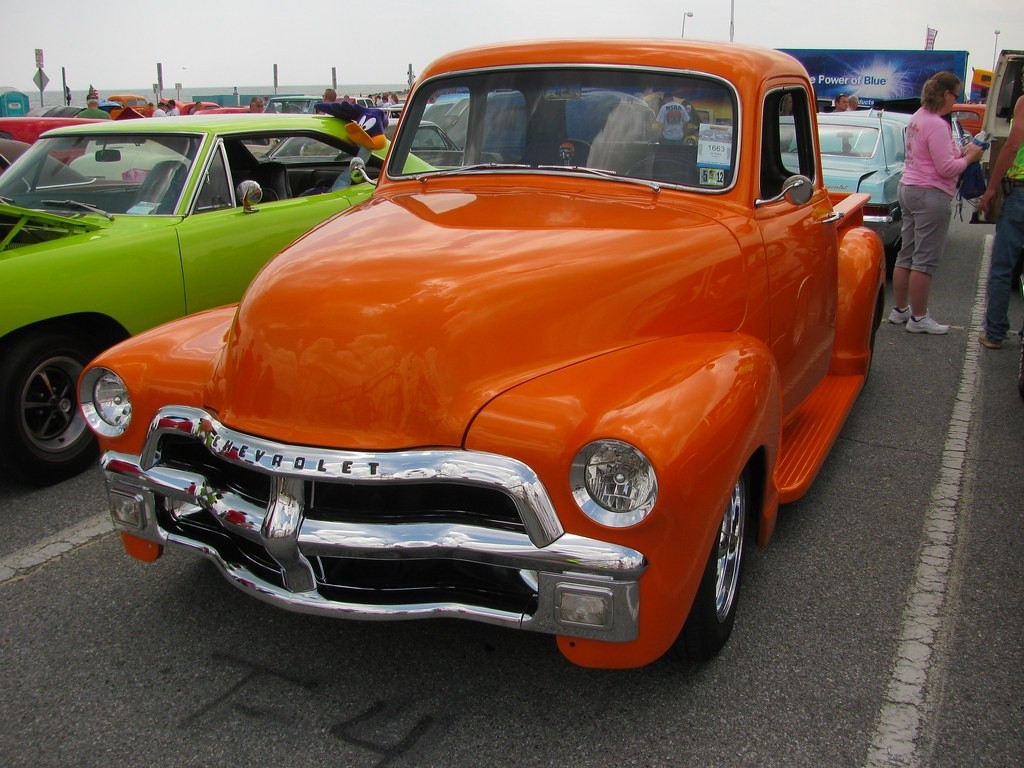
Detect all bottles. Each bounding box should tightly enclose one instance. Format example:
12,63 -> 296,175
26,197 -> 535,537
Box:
962,131 -> 986,157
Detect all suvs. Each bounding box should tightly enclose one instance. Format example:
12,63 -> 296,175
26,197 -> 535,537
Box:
969,49 -> 1024,295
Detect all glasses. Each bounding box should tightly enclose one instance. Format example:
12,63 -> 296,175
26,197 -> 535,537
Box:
839,93 -> 846,98
951,92 -> 960,101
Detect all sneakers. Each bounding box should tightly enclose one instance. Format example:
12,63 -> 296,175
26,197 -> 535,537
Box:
889,307 -> 912,323
977,332 -> 1002,348
905,307 -> 949,334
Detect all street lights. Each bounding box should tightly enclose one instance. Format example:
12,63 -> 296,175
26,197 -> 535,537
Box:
682,12 -> 693,37
992,30 -> 1000,70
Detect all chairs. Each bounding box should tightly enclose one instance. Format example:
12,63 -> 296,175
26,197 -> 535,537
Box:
132,160 -> 187,214
241,162 -> 292,203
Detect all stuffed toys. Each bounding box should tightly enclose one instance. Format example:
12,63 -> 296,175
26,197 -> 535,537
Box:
298,101 -> 389,197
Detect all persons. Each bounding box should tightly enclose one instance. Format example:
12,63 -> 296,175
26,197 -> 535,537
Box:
630,92 -> 656,141
889,71 -> 986,335
315,89 -> 355,115
779,94 -> 793,116
831,93 -> 859,112
77,94 -> 112,120
0,130 -> 15,140
656,92 -> 691,145
368,94 -> 400,118
140,100 -> 202,117
978,95 -> 1024,349
240,96 -> 275,145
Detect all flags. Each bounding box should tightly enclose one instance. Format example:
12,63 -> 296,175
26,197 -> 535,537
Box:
91,84 -> 99,98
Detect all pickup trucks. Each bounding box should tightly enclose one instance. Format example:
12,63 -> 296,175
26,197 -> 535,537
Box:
433,89 -> 735,190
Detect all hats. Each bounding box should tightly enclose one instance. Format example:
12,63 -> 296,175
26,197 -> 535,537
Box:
87,94 -> 98,99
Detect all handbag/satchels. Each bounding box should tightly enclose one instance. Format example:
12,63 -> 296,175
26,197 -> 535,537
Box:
959,161 -> 987,200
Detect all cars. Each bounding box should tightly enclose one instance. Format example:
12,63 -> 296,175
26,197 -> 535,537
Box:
263,96 -> 326,116
354,98 -> 375,108
106,95 -> 148,119
160,99 -> 220,115
0,110 -> 449,487
385,104 -> 404,118
950,103 -> 987,144
77,39 -> 887,667
259,118 -> 500,172
771,108 -> 914,265
